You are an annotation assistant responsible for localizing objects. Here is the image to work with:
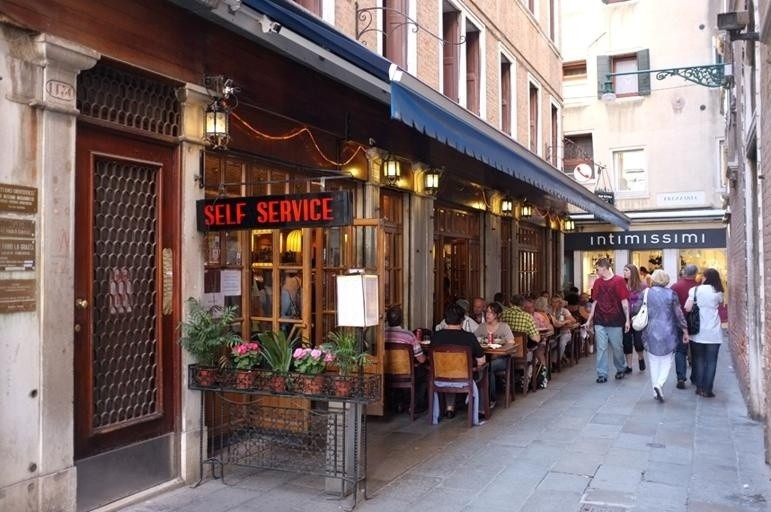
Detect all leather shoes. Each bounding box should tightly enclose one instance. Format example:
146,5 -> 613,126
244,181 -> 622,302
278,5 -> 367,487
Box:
479,410 -> 486,422
443,408 -> 456,418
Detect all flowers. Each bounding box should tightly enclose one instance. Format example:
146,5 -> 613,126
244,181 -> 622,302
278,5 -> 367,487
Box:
291,347 -> 335,375
233,342 -> 259,371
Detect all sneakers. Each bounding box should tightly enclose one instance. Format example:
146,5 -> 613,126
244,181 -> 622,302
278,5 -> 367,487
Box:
653,385 -> 665,403
695,387 -> 703,395
488,399 -> 497,408
626,367 -> 633,374
676,379 -> 686,389
615,365 -> 628,379
519,374 -> 530,392
652,389 -> 658,400
638,357 -> 647,372
702,391 -> 716,398
596,375 -> 608,383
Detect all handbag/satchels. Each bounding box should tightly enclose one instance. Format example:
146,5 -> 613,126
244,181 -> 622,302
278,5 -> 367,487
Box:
686,287 -> 701,336
631,286 -> 650,332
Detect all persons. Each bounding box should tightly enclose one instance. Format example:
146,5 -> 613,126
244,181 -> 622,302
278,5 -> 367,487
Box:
670,265 -> 703,390
622,263 -> 649,374
488,292 -> 503,315
683,268 -> 724,398
638,265 -> 650,287
631,269 -> 688,403
524,285 -> 593,387
472,303 -> 514,410
382,306 -> 425,413
500,293 -> 539,393
436,298 -> 478,333
580,258 -> 630,383
470,297 -> 487,325
429,303 -> 486,421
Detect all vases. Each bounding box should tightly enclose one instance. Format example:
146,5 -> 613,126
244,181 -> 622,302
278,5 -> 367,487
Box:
234,370 -> 254,389
299,373 -> 325,395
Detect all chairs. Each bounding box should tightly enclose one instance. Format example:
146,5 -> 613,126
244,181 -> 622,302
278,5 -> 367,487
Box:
512,331 -> 537,396
383,341 -> 424,422
427,344 -> 489,428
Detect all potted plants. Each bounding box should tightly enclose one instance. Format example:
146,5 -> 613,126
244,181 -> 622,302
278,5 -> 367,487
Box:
328,330 -> 374,399
174,297 -> 238,388
257,330 -> 297,394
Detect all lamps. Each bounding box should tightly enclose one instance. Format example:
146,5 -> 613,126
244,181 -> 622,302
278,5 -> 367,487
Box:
500,196 -> 512,213
202,96 -> 231,153
520,204 -> 532,218
564,217 -> 575,231
382,153 -> 402,188
423,167 -> 439,197
